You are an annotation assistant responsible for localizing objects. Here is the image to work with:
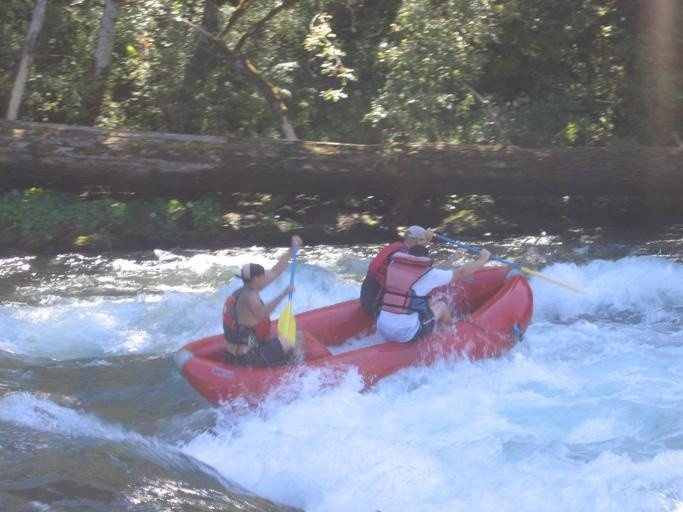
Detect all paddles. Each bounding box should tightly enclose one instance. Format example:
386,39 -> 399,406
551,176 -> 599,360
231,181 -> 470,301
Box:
430,231 -> 579,291
277,241 -> 297,343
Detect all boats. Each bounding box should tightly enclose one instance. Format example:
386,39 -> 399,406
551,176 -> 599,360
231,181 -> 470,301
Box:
169,264 -> 535,417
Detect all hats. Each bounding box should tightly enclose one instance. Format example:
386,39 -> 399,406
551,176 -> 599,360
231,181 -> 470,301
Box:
404,225 -> 427,239
241,262 -> 264,281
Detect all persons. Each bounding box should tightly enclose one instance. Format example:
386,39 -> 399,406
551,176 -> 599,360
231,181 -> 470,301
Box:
221,235 -> 330,364
375,245 -> 491,343
358,225 -> 434,319
